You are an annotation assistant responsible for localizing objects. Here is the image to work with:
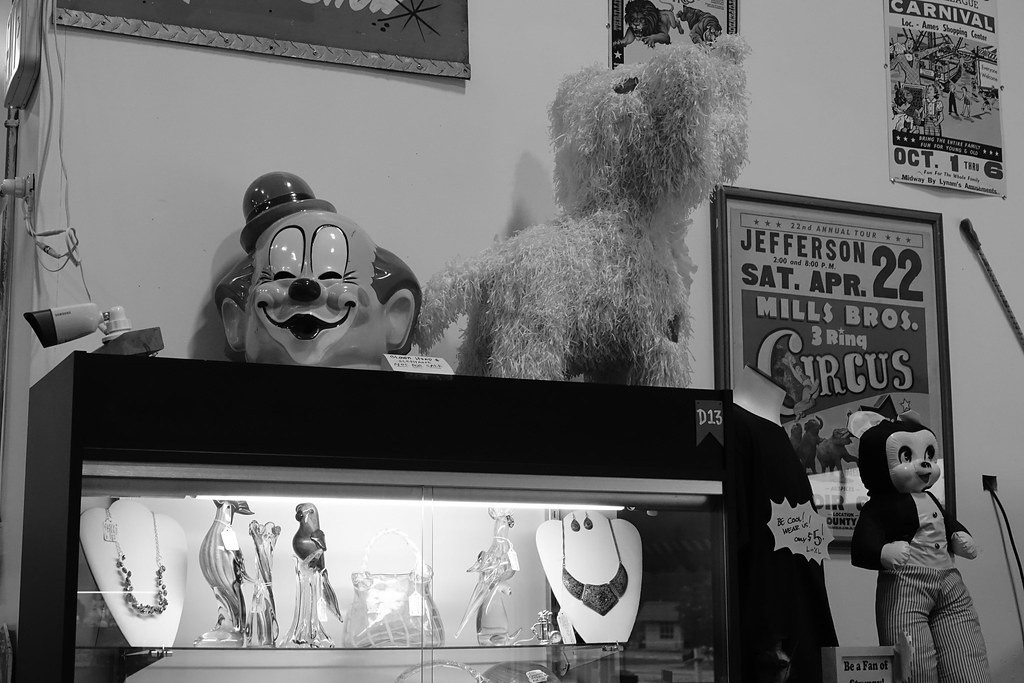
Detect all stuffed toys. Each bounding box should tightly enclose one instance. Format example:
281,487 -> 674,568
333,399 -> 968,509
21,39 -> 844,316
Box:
416,35 -> 749,388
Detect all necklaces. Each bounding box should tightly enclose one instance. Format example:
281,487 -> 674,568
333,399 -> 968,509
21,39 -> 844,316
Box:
559,515 -> 628,616
104,506 -> 169,616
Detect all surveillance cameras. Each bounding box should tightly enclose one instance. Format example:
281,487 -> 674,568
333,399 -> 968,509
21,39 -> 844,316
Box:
24,304 -> 133,348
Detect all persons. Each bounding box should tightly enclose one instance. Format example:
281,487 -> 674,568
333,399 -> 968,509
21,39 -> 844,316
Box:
724,365 -> 840,683
211,171 -> 421,370
848,394 -> 990,683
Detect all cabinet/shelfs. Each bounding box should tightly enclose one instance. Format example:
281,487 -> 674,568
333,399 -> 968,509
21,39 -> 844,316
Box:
14,350 -> 734,683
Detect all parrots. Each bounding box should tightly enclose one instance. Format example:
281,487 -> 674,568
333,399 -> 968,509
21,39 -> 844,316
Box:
291,502 -> 345,624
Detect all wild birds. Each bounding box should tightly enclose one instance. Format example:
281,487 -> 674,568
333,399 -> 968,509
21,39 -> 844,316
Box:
198,498 -> 256,631
453,506 -> 519,639
